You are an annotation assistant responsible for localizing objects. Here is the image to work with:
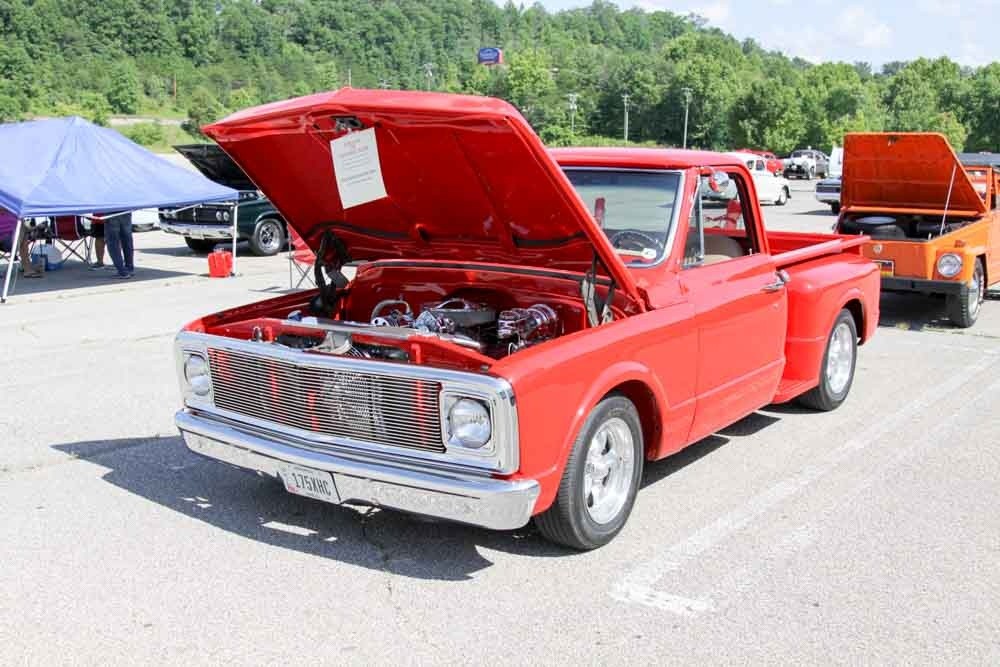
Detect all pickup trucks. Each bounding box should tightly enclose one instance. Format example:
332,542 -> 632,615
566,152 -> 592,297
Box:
168,86 -> 883,551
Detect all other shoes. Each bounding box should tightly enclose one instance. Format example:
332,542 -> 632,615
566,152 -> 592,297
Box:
111,273 -> 131,279
128,271 -> 136,276
88,264 -> 104,271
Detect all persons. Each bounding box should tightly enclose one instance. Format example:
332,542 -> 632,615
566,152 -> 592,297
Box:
88,209 -> 133,279
0,210 -> 46,276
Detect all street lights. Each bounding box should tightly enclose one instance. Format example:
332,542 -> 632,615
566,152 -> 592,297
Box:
620,88 -> 633,141
567,90 -> 577,133
679,88 -> 694,148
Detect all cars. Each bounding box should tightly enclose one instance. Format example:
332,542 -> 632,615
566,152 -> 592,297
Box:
704,152 -> 792,206
736,149 -> 783,177
158,143 -> 290,257
783,150 -> 830,180
814,142 -> 846,218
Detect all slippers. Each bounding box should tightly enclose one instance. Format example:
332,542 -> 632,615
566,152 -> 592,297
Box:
22,272 -> 44,278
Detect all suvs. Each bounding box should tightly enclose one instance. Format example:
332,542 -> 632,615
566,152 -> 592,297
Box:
831,130 -> 1000,329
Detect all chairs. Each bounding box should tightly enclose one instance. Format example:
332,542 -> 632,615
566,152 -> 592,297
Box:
0,208 -> 48,274
685,233 -> 744,258
594,198 -> 606,229
285,222 -> 317,289
704,199 -> 742,230
53,216 -> 94,266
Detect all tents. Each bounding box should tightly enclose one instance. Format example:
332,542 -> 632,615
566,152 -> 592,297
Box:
0,115 -> 240,302
